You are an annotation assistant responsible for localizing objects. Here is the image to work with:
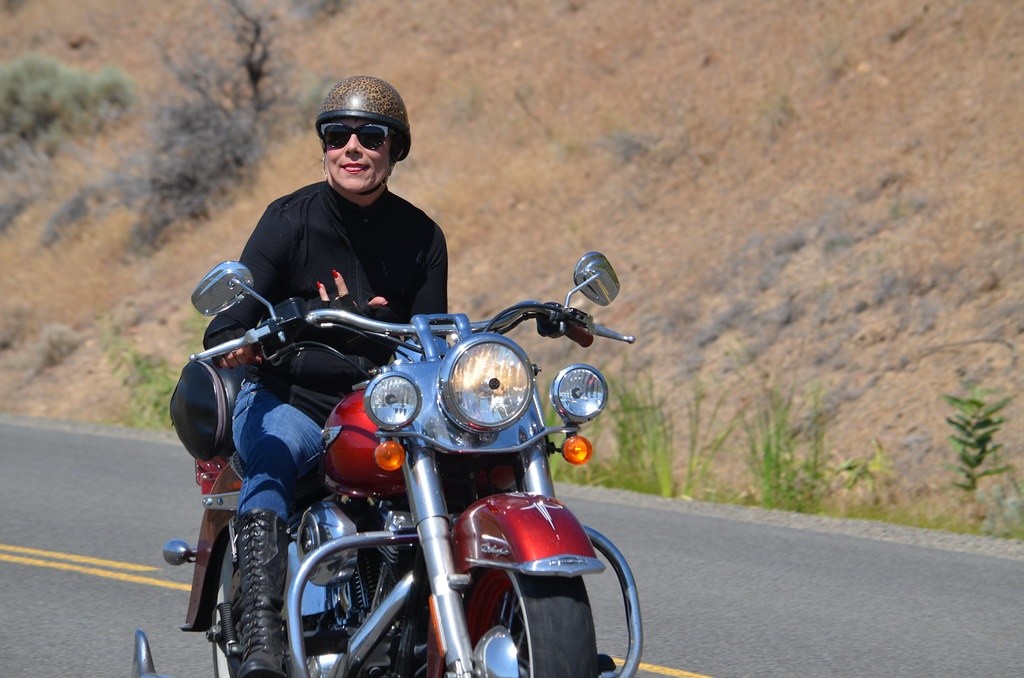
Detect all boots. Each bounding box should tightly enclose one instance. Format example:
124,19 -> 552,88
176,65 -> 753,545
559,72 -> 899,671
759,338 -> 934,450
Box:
233,506 -> 292,678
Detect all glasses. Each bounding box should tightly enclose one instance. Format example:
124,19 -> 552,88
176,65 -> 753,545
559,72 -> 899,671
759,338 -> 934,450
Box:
318,120 -> 396,150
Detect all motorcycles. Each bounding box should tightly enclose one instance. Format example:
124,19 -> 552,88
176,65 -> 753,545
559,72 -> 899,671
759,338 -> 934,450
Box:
132,252 -> 644,678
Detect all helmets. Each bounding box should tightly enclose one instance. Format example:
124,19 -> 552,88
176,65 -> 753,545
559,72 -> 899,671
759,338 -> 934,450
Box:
312,75 -> 412,161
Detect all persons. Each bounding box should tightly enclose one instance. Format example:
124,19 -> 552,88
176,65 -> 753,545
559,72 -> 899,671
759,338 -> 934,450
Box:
203,74 -> 448,678
